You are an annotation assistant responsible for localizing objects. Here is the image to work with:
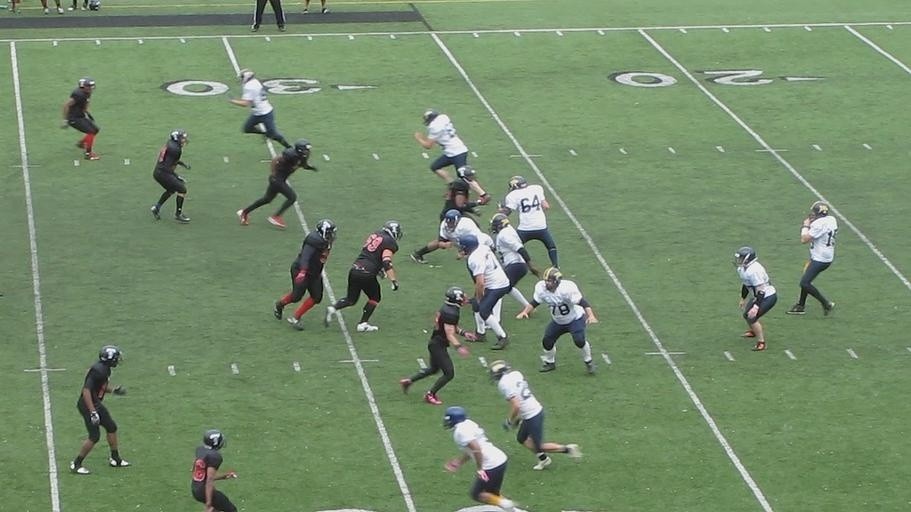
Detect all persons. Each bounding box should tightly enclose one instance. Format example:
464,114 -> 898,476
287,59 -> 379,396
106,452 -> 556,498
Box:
67,0 -> 89,11
152,128 -> 191,224
786,200 -> 837,319
192,430 -> 238,512
41,0 -> 64,13
71,344 -> 131,474
250,0 -> 286,31
303,0 -> 329,14
734,246 -> 777,351
61,76 -> 100,161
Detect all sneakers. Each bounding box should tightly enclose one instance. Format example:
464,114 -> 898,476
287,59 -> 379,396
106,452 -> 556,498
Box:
151,205 -> 192,224
400,377 -> 413,394
324,306 -> 339,330
8,6 -> 92,16
740,328 -> 768,352
785,304 -> 806,315
532,457 -> 551,471
424,390 -> 442,404
411,250 -> 428,264
76,140 -> 100,161
538,361 -> 558,374
273,301 -> 305,333
582,361 -> 599,375
235,209 -> 250,226
250,7 -> 331,31
462,329 -> 510,352
267,214 -> 285,229
823,301 -> 835,316
69,460 -> 90,475
109,457 -> 130,468
566,443 -> 582,461
354,319 -> 382,333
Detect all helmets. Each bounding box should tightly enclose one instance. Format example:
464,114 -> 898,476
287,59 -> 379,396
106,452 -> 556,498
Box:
487,359 -> 510,379
382,220 -> 403,240
294,139 -> 312,157
316,219 -> 338,242
98,344 -> 120,366
443,405 -> 467,430
423,109 -> 442,127
734,247 -> 756,266
170,127 -> 189,146
239,69 -> 255,85
438,164 -> 563,308
202,427 -> 225,450
809,202 -> 831,217
79,78 -> 96,90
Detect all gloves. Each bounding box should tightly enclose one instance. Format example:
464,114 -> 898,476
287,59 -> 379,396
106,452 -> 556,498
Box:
380,271 -> 387,279
60,119 -> 69,129
444,457 -> 460,471
503,417 -> 520,431
89,411 -> 102,426
475,470 -> 489,484
295,270 -> 306,283
391,279 -> 399,292
112,383 -> 128,396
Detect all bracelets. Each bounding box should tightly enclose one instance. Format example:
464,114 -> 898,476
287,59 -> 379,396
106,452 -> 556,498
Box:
800,225 -> 811,231
